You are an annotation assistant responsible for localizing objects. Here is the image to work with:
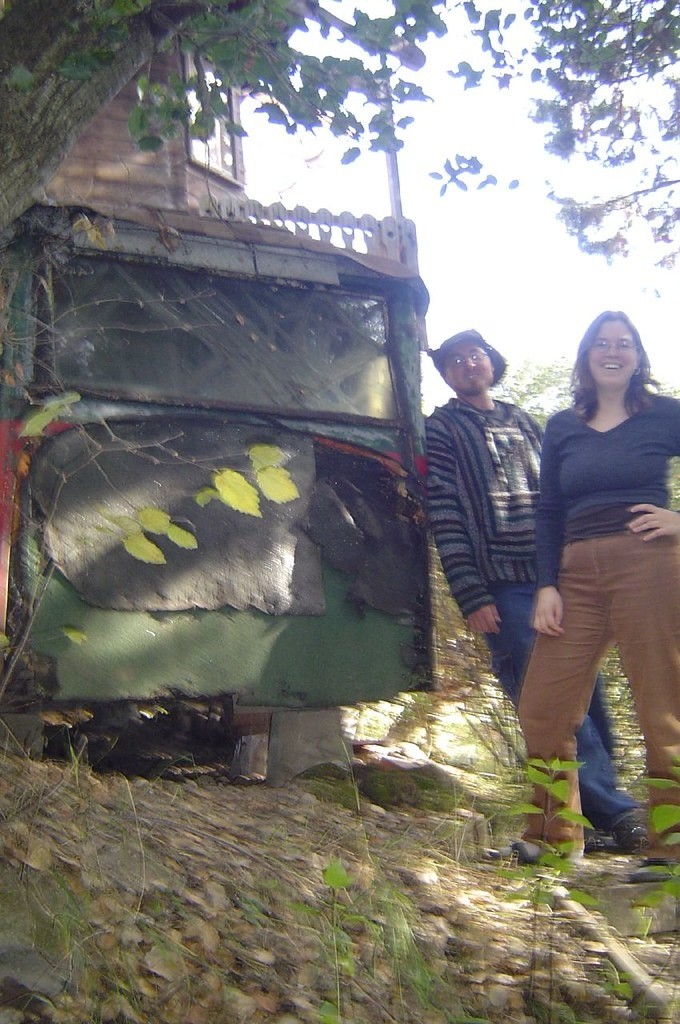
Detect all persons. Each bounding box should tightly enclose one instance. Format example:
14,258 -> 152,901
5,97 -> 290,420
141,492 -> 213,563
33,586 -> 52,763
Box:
424,328 -> 647,853
512,311 -> 679,881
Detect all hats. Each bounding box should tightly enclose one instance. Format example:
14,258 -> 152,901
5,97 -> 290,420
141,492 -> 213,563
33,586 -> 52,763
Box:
433,330 -> 507,386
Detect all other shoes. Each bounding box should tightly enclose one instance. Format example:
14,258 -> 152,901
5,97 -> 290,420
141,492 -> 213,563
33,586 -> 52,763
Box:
482,840 -> 541,865
629,861 -> 680,883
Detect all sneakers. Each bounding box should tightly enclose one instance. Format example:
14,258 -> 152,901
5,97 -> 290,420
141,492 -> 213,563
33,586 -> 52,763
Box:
582,826 -> 618,852
612,808 -> 650,854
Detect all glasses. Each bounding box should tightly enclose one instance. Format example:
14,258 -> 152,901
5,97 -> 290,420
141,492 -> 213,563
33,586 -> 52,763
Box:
441,353 -> 488,368
593,338 -> 638,352
